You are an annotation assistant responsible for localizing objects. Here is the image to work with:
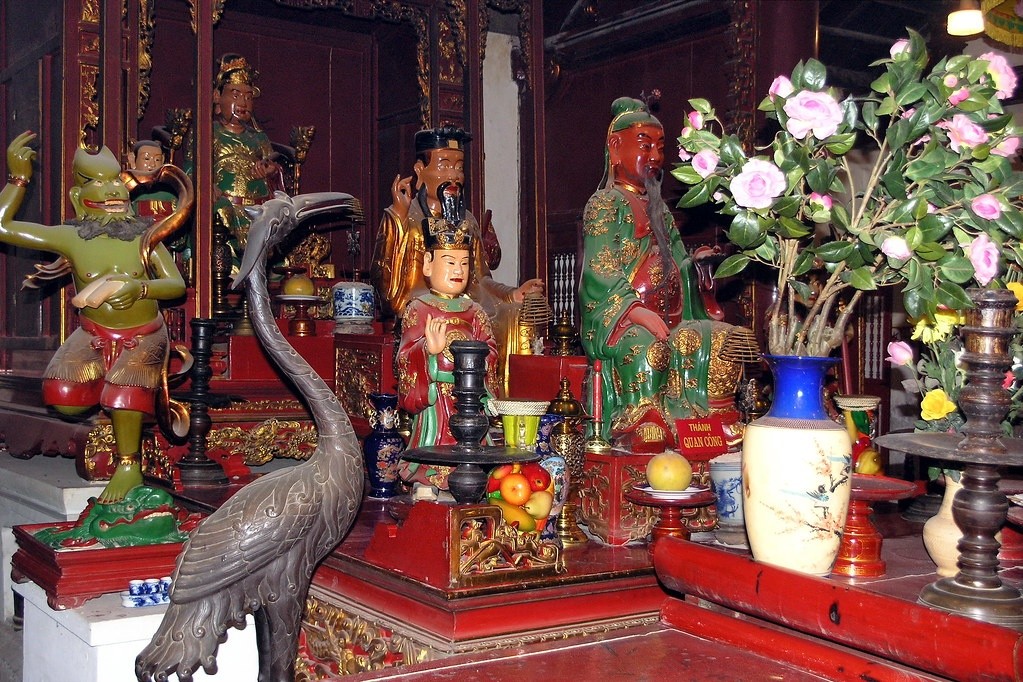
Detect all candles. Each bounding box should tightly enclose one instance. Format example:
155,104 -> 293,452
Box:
592,359 -> 602,419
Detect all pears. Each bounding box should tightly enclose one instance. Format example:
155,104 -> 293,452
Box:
524,491 -> 553,519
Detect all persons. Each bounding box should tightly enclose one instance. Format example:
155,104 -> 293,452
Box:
118,141 -> 166,226
371,123 -> 546,323
395,217 -> 500,500
580,97 -> 729,443
0,130 -> 186,503
184,52 -> 295,289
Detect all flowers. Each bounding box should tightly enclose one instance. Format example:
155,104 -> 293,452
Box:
673,27 -> 1023,359
884,281 -> 1023,481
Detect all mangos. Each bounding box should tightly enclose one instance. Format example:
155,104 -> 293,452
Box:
488,498 -> 536,533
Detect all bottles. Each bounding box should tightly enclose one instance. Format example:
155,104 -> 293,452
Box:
364,393 -> 405,499
486,399 -> 555,544
834,395 -> 881,474
536,414 -> 571,550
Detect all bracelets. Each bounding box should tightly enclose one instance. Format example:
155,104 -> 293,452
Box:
7,175 -> 30,188
141,280 -> 148,300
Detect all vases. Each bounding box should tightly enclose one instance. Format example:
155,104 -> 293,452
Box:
365,393 -> 406,501
744,352 -> 853,579
923,471 -> 1000,577
536,415 -> 570,550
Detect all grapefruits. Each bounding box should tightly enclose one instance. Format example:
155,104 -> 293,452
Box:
645,452 -> 692,491
283,275 -> 314,296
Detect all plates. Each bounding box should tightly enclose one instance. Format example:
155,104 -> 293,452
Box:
632,484 -> 710,499
275,295 -> 321,300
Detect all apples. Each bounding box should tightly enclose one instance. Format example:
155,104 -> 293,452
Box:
486,463 -> 551,506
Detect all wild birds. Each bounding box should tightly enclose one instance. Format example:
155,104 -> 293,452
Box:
133,192 -> 363,681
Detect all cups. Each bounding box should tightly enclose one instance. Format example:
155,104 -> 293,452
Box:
145,579 -> 160,594
129,580 -> 147,596
160,577 -> 172,593
